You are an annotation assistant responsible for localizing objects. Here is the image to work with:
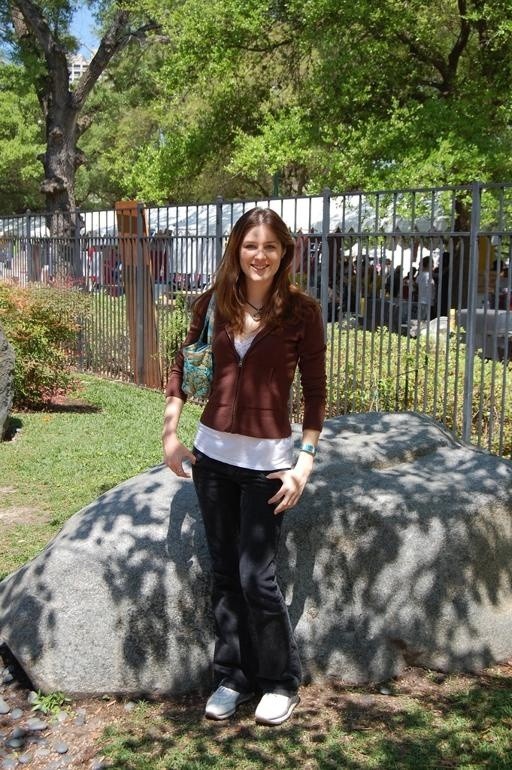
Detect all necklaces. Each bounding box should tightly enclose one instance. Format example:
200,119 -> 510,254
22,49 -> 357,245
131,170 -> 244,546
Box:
247,302 -> 266,321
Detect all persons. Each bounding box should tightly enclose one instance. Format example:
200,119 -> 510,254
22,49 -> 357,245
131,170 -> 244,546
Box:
162,207 -> 327,726
342,252 -> 508,320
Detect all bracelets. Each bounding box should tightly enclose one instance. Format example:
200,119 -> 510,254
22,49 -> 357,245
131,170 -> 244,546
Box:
300,443 -> 316,457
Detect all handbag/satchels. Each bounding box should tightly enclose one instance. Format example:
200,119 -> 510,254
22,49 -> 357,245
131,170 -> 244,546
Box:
180,341 -> 214,399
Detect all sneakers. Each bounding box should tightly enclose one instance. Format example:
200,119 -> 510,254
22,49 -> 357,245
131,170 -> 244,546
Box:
253,689 -> 300,724
203,686 -> 256,720
